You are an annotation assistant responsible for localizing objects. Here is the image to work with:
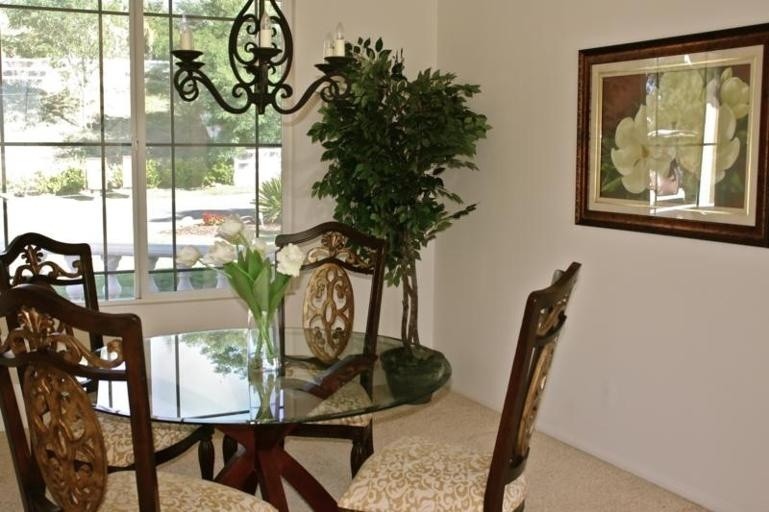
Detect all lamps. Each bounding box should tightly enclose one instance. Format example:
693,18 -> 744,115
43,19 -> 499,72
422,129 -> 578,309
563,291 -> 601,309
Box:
169,0 -> 356,119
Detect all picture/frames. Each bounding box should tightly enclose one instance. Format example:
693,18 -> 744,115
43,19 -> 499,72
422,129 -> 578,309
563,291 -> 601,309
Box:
573,21 -> 769,251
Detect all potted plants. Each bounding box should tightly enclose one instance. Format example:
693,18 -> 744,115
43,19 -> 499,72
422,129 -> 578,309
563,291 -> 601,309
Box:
307,34 -> 496,403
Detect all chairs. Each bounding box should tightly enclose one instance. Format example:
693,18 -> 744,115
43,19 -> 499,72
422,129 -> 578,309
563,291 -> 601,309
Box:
221,222 -> 391,490
335,258 -> 585,512
0,220 -> 215,482
1,280 -> 287,512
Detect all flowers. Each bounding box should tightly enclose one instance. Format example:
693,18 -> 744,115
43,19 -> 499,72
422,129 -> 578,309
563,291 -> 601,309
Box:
176,212 -> 303,361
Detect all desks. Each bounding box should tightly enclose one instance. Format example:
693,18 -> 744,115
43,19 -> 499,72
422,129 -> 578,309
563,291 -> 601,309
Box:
72,325 -> 454,512
